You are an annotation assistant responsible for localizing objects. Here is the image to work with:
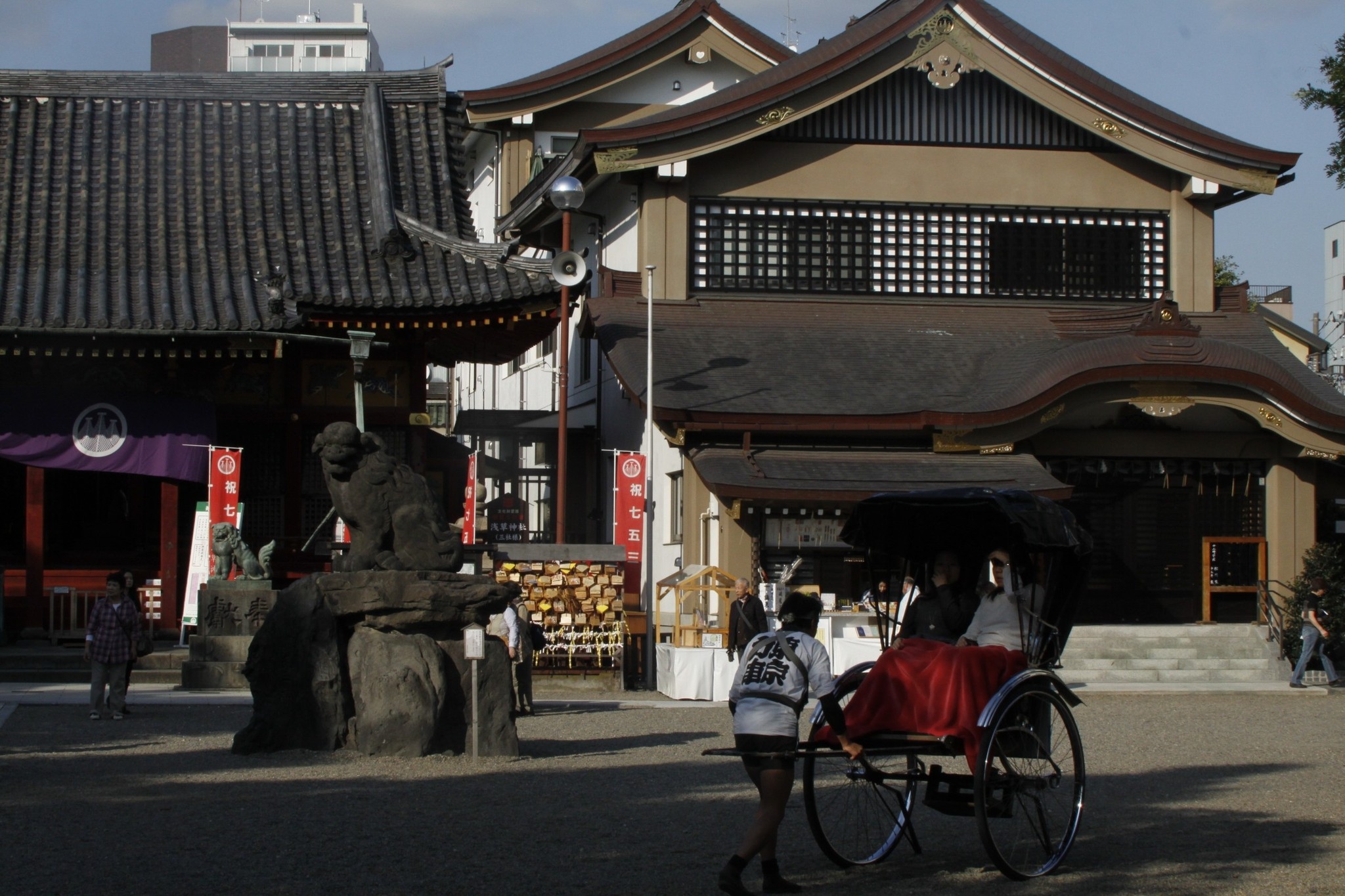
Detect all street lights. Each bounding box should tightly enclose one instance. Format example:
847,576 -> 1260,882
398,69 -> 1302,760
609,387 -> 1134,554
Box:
547,175 -> 586,544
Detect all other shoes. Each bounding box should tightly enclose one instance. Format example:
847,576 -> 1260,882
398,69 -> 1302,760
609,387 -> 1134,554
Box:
106,702 -> 131,714
515,706 -> 535,717
718,872 -> 754,896
90,713 -> 100,720
1329,679 -> 1345,687
763,876 -> 803,894
112,714 -> 123,720
1290,683 -> 1307,688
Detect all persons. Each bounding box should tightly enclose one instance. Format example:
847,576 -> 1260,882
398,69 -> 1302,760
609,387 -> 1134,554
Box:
1290,577 -> 1345,688
861,580 -> 887,602
728,578 -> 768,662
714,593 -> 866,895
895,575 -> 920,636
486,581 -> 535,714
84,570 -> 144,719
891,540 -> 1044,651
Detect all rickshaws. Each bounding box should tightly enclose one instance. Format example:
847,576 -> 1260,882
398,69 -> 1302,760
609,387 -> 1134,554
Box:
700,486 -> 1088,883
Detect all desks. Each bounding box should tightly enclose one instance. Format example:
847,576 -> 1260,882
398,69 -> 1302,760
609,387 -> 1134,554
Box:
767,611 -> 886,682
656,644 -> 741,701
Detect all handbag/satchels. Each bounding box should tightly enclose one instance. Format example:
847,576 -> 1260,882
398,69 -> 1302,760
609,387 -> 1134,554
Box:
136,637 -> 154,658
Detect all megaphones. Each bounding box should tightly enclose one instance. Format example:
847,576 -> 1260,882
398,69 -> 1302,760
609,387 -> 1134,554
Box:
551,250 -> 586,287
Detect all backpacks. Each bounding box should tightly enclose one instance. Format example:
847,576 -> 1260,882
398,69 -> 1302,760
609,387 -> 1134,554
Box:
518,603 -> 546,651
